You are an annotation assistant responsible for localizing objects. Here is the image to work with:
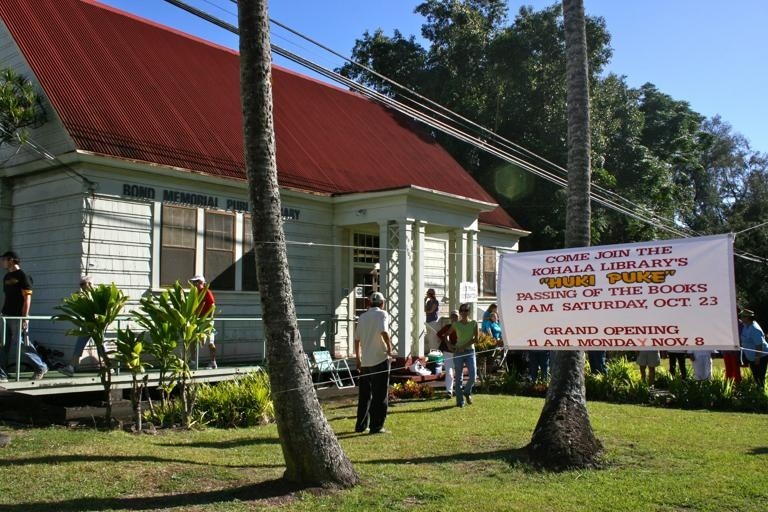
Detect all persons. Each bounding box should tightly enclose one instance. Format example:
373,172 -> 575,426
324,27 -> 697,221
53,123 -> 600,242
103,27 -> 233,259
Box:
187,274 -> 218,369
482,304 -> 497,331
686,350 -> 719,381
668,350 -> 687,379
486,311 -> 501,339
587,350 -> 607,374
738,308 -> 768,384
445,304 -> 479,406
57,274 -> 116,377
637,351 -> 660,385
352,291 -> 392,433
423,287 -> 439,350
528,350 -> 549,384
0,250 -> 49,382
437,309 -> 459,398
723,350 -> 743,383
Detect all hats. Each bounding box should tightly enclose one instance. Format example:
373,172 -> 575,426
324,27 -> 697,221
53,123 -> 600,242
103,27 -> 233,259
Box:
451,310 -> 459,317
370,292 -> 386,303
0,251 -> 18,258
189,274 -> 205,282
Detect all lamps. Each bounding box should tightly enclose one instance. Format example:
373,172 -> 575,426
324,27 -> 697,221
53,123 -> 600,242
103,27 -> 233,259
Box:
355,209 -> 367,215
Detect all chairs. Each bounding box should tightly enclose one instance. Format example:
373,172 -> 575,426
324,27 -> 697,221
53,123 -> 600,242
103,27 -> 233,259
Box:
313,350 -> 355,390
305,354 -> 313,381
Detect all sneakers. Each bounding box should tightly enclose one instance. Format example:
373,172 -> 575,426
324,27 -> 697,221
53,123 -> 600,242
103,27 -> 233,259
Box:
356,427 -> 392,435
0,377 -> 8,382
187,359 -> 217,369
101,368 -> 115,377
457,392 -> 472,408
57,365 -> 74,377
32,366 -> 48,381
447,393 -> 452,399
